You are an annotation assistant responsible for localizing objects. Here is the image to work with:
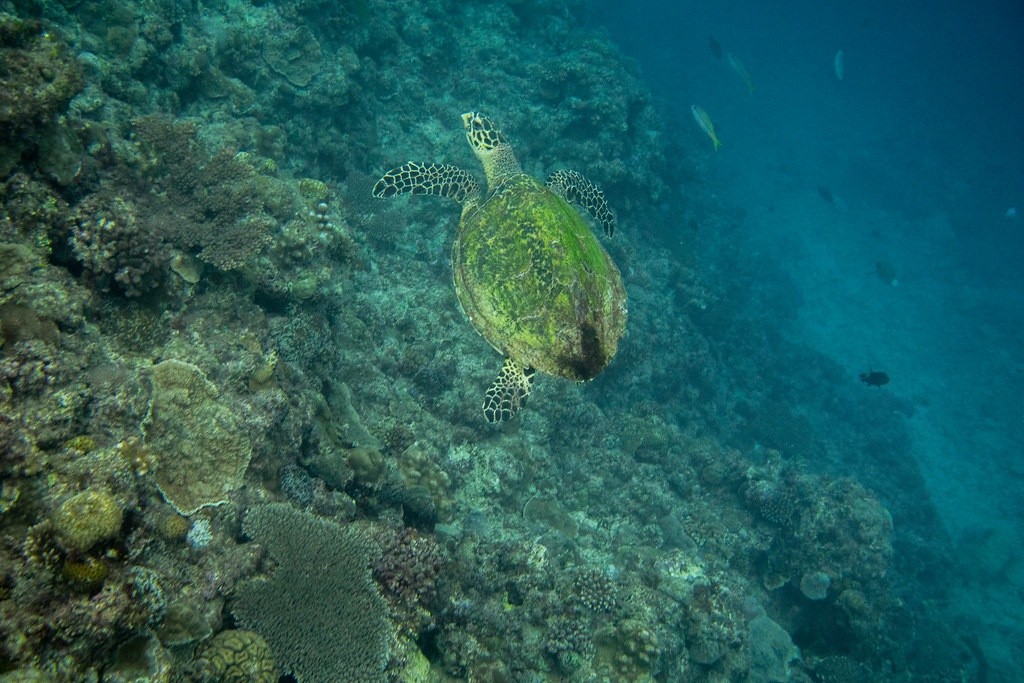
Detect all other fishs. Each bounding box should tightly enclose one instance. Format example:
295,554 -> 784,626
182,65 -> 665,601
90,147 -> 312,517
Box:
692,34 -> 756,152
859,367 -> 889,389
